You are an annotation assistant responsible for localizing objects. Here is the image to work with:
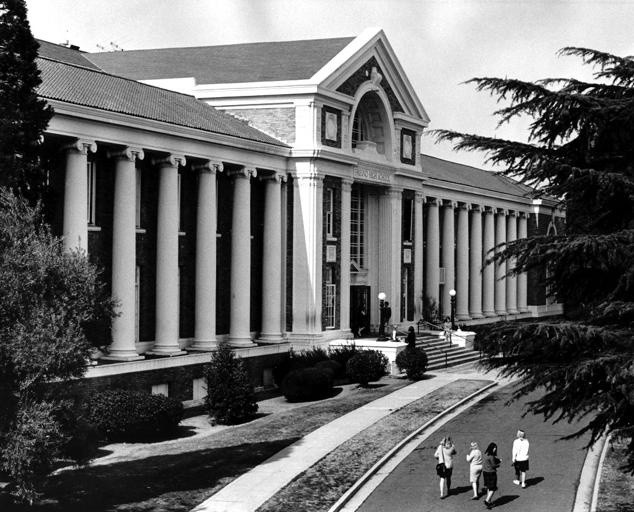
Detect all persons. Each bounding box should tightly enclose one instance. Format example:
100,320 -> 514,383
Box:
443,316 -> 453,347
357,303 -> 368,337
382,301 -> 391,327
434,436 -> 457,500
466,441 -> 483,500
481,442 -> 502,508
511,430 -> 530,488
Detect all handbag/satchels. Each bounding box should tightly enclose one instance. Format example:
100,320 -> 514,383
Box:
436,463 -> 447,478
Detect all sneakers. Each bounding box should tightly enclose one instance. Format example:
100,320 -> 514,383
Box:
470,496 -> 479,500
521,483 -> 527,489
440,496 -> 444,499
513,479 -> 520,486
482,500 -> 496,510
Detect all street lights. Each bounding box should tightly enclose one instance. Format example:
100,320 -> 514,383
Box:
377,292 -> 389,342
448,288 -> 458,332
